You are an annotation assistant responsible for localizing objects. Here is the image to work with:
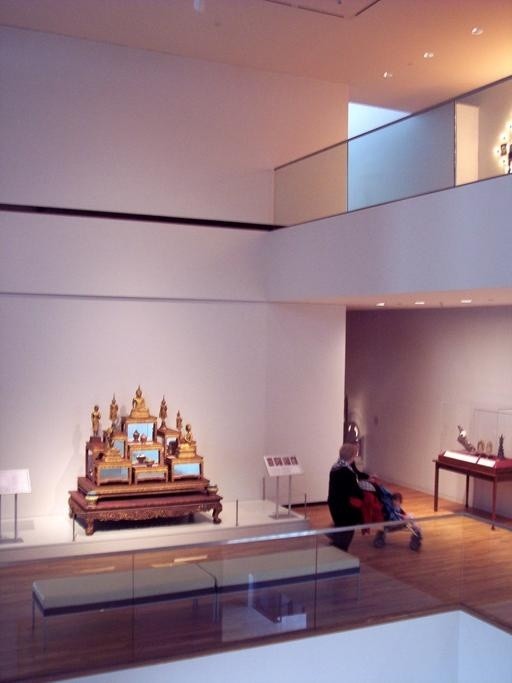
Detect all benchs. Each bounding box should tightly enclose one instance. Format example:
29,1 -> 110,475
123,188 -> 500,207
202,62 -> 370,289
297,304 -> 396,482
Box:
195,546 -> 361,623
31,563 -> 217,650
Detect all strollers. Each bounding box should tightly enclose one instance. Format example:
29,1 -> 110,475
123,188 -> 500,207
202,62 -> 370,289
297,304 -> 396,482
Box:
364,473 -> 424,553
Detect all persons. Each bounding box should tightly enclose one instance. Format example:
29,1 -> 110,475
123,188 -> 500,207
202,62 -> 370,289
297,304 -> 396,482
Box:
391,493 -> 423,531
327,443 -> 378,552
